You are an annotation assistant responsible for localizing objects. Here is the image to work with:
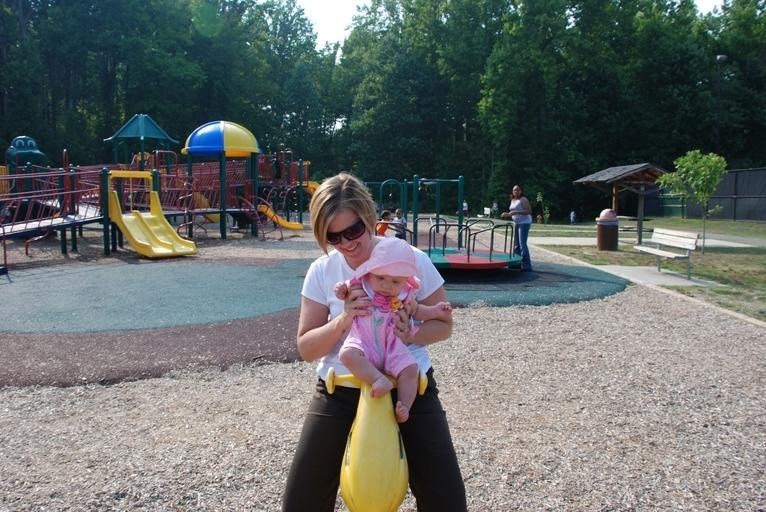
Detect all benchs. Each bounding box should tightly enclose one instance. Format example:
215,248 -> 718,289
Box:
632,227 -> 701,280
477,206 -> 492,219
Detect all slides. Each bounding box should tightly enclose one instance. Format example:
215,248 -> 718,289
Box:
303,180 -> 320,195
257,205 -> 304,229
107,170 -> 198,259
174,177 -> 224,223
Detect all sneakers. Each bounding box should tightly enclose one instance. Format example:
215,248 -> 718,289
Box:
520,267 -> 532,273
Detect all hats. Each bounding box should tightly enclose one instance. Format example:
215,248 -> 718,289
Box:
353,234 -> 421,282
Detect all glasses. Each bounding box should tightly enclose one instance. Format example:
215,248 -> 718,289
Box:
324,218 -> 366,246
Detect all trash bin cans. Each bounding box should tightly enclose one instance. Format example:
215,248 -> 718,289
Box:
595,208 -> 618,251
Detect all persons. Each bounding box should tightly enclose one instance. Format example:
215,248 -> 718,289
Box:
462,198 -> 469,217
388,208 -> 408,242
282,169 -> 471,511
499,184 -> 536,273
490,201 -> 498,217
332,236 -> 454,423
375,209 -> 392,237
569,208 -> 577,226
